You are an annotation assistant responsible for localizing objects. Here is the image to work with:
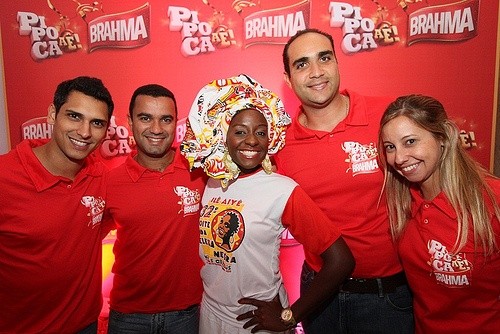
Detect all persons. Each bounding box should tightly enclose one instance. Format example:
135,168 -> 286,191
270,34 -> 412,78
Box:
270,28 -> 419,334
102,84 -> 207,334
178,74 -> 356,334
0,76 -> 112,333
375,94 -> 499,333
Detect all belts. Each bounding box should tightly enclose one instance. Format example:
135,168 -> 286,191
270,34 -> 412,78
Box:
342,271 -> 409,294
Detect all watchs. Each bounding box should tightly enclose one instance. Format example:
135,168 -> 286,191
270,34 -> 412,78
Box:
281,306 -> 298,327
